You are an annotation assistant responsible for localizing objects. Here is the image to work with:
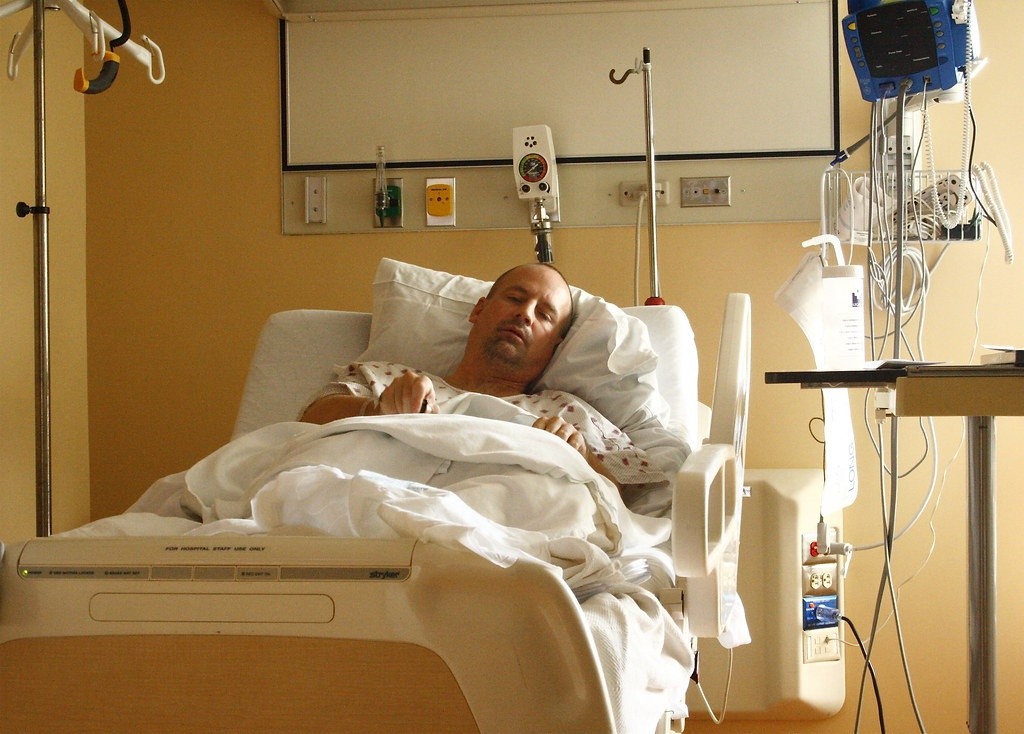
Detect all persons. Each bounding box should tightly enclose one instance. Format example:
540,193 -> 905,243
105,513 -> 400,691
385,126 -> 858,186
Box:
298,263 -> 670,507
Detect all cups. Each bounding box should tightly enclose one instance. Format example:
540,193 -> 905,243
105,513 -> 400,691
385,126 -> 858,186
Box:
822,265 -> 865,370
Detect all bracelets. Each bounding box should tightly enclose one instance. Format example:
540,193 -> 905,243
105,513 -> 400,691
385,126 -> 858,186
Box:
359,397 -> 376,416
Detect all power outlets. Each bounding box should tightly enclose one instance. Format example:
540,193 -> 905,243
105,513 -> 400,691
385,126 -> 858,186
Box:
620,181 -> 670,207
803,560 -> 838,594
427,177 -> 457,226
802,530 -> 840,564
680,175 -> 732,209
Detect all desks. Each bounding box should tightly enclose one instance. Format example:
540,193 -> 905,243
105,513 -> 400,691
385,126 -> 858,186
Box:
894,377 -> 1024,733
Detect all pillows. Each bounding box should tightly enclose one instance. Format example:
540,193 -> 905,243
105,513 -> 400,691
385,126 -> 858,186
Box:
360,258 -> 666,430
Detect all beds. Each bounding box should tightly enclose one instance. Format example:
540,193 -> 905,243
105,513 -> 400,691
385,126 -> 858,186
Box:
0,292 -> 762,733
